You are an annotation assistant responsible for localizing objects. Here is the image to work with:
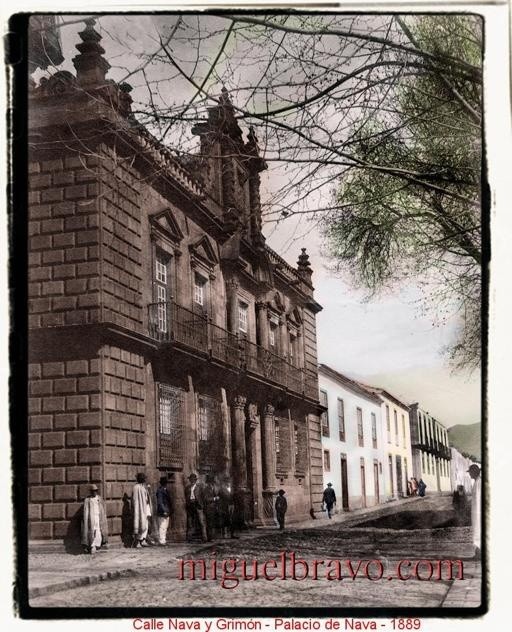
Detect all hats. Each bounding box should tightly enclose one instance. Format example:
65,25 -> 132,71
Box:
88,484 -> 99,491
206,475 -> 215,480
466,464 -> 482,473
135,472 -> 147,479
279,489 -> 286,494
327,483 -> 332,486
187,474 -> 197,480
158,476 -> 169,483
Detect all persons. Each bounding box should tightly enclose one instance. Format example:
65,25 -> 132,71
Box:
453,484 -> 467,522
183,471 -> 248,543
466,464 -> 482,560
407,476 -> 427,497
274,489 -> 287,530
80,483 -> 108,556
322,482 -> 337,519
128,473 -> 150,549
155,476 -> 172,547
